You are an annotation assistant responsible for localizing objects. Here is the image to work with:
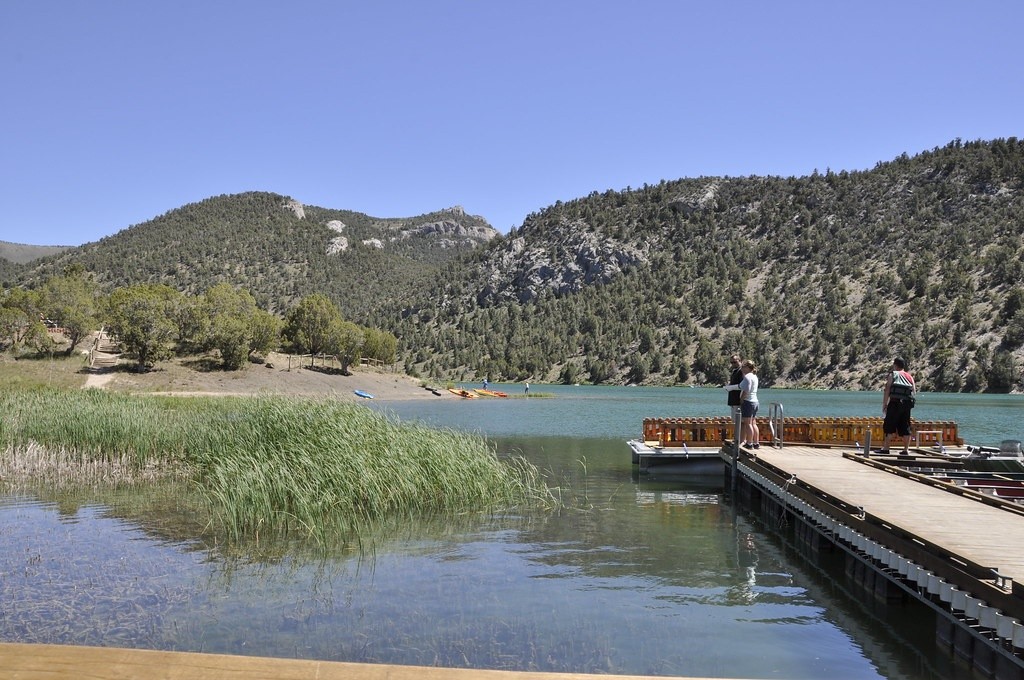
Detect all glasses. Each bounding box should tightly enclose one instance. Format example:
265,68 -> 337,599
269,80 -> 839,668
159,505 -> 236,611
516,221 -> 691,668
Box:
730,360 -> 738,364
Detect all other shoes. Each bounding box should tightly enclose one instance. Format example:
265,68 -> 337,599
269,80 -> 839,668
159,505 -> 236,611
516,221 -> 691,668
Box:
753,443 -> 760,449
899,450 -> 908,455
743,443 -> 752,449
874,449 -> 890,454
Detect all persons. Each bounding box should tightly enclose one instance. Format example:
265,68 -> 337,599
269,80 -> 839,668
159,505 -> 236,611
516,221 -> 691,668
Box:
482,378 -> 488,390
525,381 -> 529,394
723,353 -> 747,445
740,359 -> 760,449
874,357 -> 915,455
